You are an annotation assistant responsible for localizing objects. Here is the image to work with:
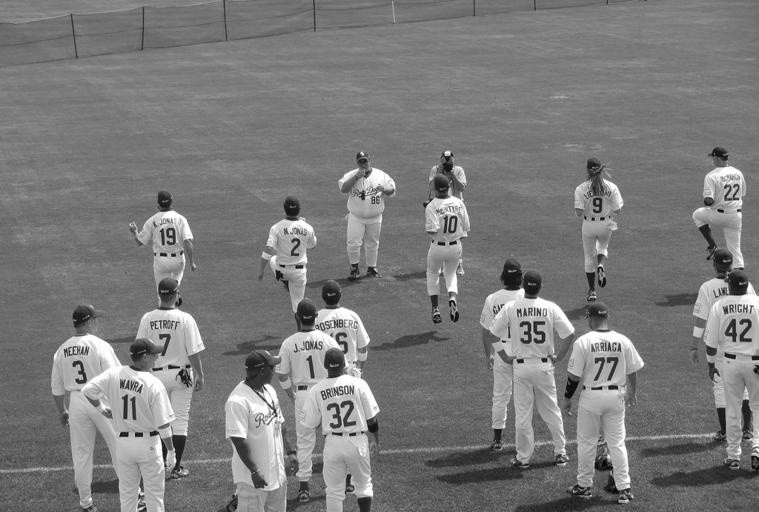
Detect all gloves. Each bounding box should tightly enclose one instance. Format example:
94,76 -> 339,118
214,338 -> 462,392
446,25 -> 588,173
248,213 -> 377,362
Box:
96,403 -> 112,417
166,449 -> 176,472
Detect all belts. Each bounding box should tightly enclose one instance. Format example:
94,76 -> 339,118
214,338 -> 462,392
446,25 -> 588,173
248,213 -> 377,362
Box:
432,240 -> 457,246
298,386 -> 308,390
152,364 -> 191,370
516,358 -> 548,363
119,431 -> 159,437
332,431 -> 364,436
583,385 -> 618,390
717,209 -> 742,213
584,216 -> 611,220
724,353 -> 759,361
279,265 -> 303,269
153,252 -> 183,256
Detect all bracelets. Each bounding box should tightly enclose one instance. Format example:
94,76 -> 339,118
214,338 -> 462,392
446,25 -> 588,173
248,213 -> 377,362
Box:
691,347 -> 699,351
706,354 -> 717,365
95,403 -> 106,413
287,449 -> 296,455
492,341 -> 504,353
251,468 -> 261,475
260,250 -> 272,262
564,377 -> 579,400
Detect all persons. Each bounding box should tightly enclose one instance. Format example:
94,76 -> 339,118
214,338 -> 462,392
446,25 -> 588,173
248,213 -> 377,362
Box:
573,156 -> 624,301
689,247 -> 757,443
81,338 -> 177,511
315,280 -> 370,378
299,347 -> 379,511
704,271 -> 759,472
257,196 -> 318,331
51,304 -> 146,510
489,269 -> 575,469
424,173 -> 471,324
276,298 -> 356,502
691,146 -> 747,271
224,350 -> 299,510
127,189 -> 198,310
338,150 -> 397,280
562,301 -> 644,505
479,257 -> 526,451
136,278 -> 205,479
423,150 -> 467,275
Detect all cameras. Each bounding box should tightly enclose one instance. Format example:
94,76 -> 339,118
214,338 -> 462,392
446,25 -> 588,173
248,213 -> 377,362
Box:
443,163 -> 452,171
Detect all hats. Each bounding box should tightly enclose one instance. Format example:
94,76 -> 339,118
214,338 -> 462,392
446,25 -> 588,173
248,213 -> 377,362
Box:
357,151 -> 370,162
434,174 -> 449,196
503,258 -> 522,285
587,158 -> 601,169
158,191 -> 173,207
584,301 -> 608,319
708,147 -> 728,157
324,348 -> 345,371
245,350 -> 281,371
322,280 -> 341,305
73,304 -> 106,324
158,278 -> 180,295
523,270 -> 542,295
725,270 -> 748,295
284,196 -> 299,216
297,297 -> 316,326
130,338 -> 164,354
713,249 -> 733,271
441,151 -> 455,163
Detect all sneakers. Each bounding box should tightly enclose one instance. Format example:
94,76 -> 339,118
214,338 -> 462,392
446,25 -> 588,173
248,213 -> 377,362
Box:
432,306 -> 442,323
84,505 -> 97,512
171,466 -> 189,480
713,427 -> 759,472
367,268 -> 382,278
298,490 -> 310,503
706,245 -> 717,260
137,492 -> 147,511
348,269 -> 360,281
449,297 -> 459,322
346,485 -> 355,495
597,264 -> 606,288
490,441 -> 635,506
587,289 -> 596,301
